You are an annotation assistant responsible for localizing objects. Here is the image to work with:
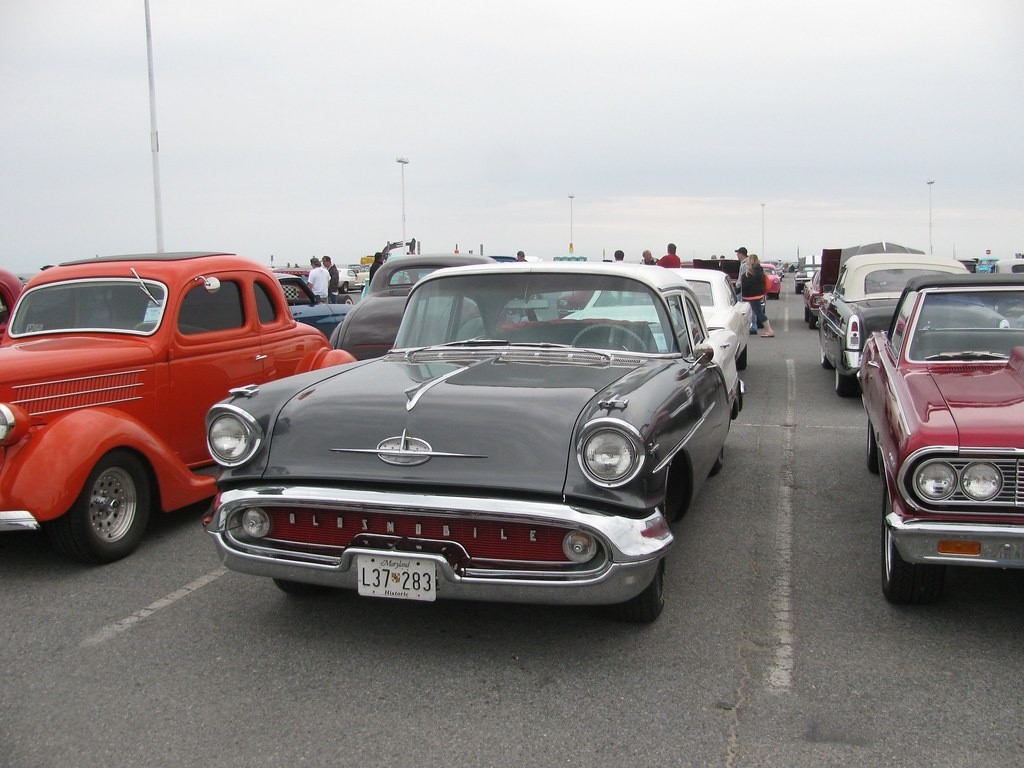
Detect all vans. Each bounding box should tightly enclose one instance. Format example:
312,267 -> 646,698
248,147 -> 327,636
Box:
973,257 -> 999,275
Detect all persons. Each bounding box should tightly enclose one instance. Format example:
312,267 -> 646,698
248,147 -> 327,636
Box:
641,250 -> 659,265
655,244 -> 681,268
369,252 -> 383,287
320,256 -> 339,304
294,263 -> 299,268
735,247 -> 758,335
307,258 -> 331,304
515,251 -> 527,262
741,254 -> 776,338
614,250 -> 625,263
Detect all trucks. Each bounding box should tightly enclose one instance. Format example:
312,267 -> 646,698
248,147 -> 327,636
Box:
788,261 -> 799,272
799,255 -> 822,272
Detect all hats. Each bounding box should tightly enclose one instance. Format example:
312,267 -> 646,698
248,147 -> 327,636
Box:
735,247 -> 747,252
310,256 -> 319,265
319,256 -> 330,262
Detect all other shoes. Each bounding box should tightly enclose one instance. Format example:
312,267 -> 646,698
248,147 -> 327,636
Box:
761,334 -> 775,337
757,325 -> 764,328
750,329 -> 757,335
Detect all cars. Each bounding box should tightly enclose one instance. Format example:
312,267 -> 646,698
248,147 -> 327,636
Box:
857,272 -> 1024,607
759,263 -> 784,300
336,269 -> 366,296
328,253 -> 499,362
0,250 -> 356,568
563,268 -> 753,371
271,271 -> 357,343
794,271 -> 817,295
1,268 -> 23,337
818,253 -> 1011,398
203,260 -> 732,626
803,270 -> 824,330
693,258 -> 741,287
995,259 -> 1024,274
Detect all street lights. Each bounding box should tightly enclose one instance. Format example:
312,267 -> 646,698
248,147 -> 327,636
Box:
761,202 -> 766,261
568,194 -> 574,257
927,181 -> 935,253
395,157 -> 411,255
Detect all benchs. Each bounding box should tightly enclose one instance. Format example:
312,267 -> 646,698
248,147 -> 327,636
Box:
490,318 -> 662,354
913,327 -> 1024,360
